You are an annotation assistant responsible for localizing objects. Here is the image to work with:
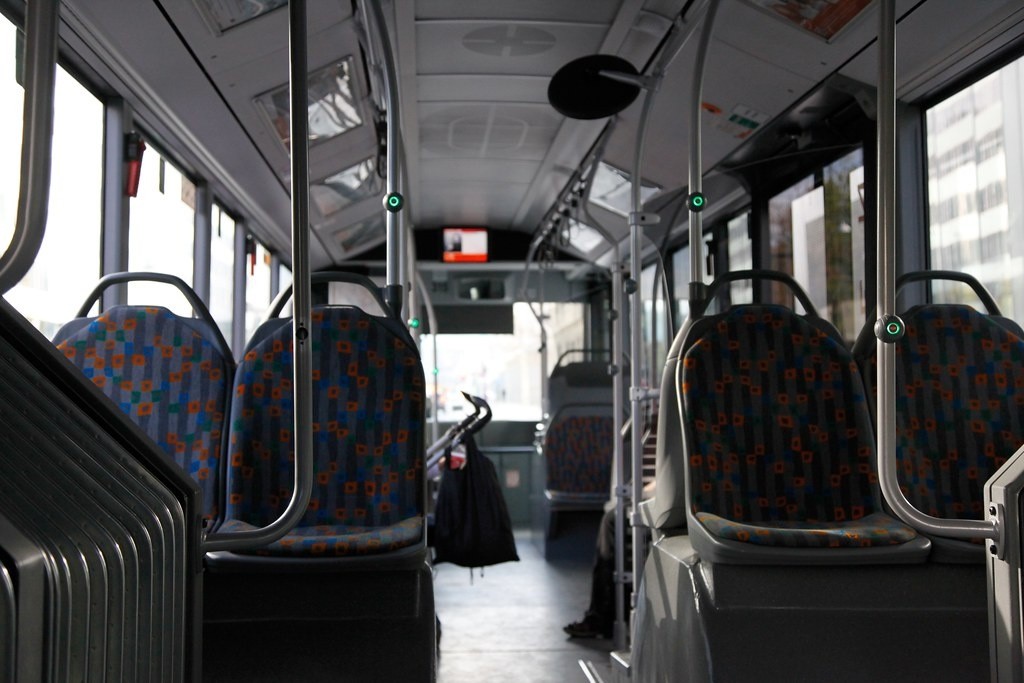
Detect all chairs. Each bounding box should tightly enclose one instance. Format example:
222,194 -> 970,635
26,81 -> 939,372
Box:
53,269 -> 1024,683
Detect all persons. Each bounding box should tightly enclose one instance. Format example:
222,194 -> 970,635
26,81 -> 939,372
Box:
562,482 -> 655,639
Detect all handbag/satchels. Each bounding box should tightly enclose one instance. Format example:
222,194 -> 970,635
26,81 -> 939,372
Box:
432,432 -> 520,568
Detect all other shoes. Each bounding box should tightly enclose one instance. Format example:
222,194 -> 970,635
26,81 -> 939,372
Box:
563,611 -> 613,639
